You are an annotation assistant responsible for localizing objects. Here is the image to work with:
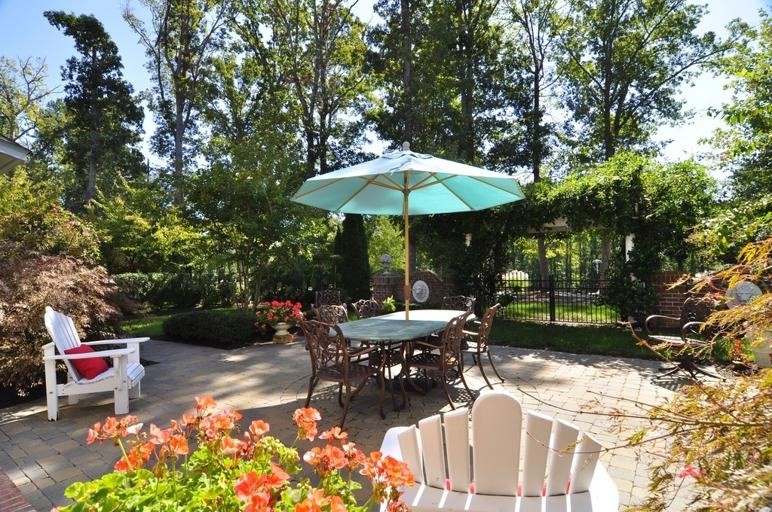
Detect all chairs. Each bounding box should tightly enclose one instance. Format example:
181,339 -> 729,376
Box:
38,306 -> 150,421
311,304 -> 362,372
296,319 -> 385,428
410,309 -> 475,410
311,289 -> 341,320
644,298 -> 727,385
462,302 -> 504,389
370,393 -> 618,512
350,299 -> 392,361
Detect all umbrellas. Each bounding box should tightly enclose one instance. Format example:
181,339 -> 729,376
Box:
289,142 -> 527,380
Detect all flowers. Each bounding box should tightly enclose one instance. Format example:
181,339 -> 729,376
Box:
251,300 -> 307,329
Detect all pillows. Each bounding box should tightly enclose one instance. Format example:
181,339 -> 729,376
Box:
445,477 -> 571,497
63,344 -> 110,379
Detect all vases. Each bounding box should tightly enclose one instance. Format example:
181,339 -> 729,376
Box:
268,322 -> 293,344
